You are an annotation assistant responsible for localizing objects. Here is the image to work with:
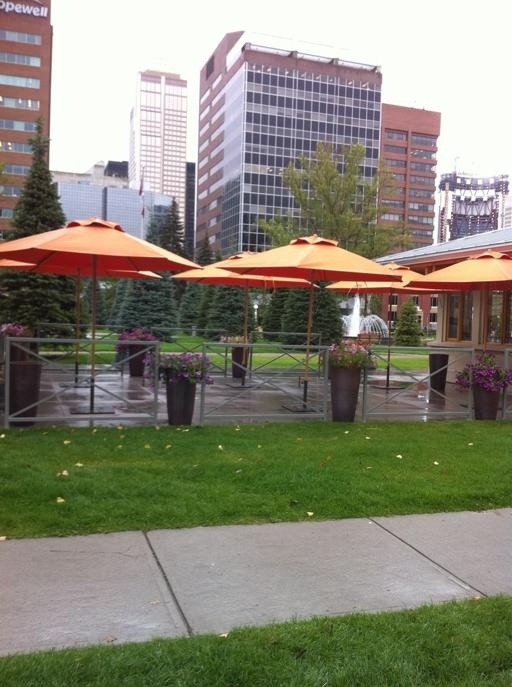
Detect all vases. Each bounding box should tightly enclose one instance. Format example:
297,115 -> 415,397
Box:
165,380 -> 195,426
430,352 -> 448,390
232,346 -> 247,379
330,363 -> 362,421
7,339 -> 41,427
129,342 -> 148,376
476,385 -> 498,419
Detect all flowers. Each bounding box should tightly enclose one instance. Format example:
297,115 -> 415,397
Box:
114,326 -> 160,351
141,348 -> 216,393
454,347 -> 512,394
326,339 -> 377,372
2,320 -> 25,336
219,333 -> 250,346
